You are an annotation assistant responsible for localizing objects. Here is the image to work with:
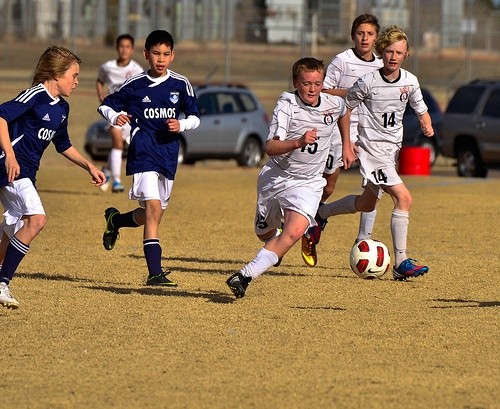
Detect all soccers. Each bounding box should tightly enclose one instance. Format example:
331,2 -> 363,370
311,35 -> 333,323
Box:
350,238 -> 391,280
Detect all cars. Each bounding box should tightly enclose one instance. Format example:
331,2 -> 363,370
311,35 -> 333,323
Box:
442,78 -> 500,178
340,86 -> 441,172
83,82 -> 271,168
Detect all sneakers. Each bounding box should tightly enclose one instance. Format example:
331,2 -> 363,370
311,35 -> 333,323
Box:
392,257 -> 429,281
273,222 -> 285,268
100,167 -> 110,193
226,272 -> 252,298
112,183 -> 124,191
301,235 -> 317,267
309,202 -> 328,244
146,272 -> 177,286
0,282 -> 20,310
103,207 -> 120,250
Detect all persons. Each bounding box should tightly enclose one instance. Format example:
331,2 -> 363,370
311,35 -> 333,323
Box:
225,57 -> 357,299
95,29 -> 203,289
301,13 -> 435,282
98,33 -> 146,192
0,46 -> 108,308
